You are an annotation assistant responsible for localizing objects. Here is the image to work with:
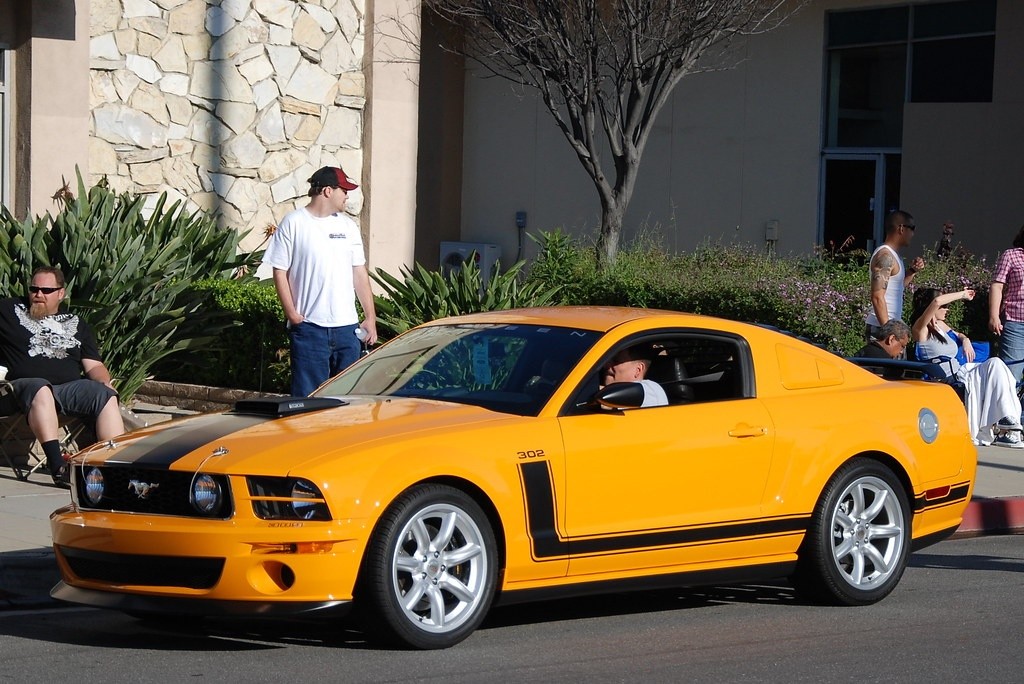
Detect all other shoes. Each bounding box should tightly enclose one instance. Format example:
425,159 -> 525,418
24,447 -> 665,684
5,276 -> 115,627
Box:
52,464 -> 72,488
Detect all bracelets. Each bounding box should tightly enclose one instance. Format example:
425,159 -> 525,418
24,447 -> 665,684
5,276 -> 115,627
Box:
909,267 -> 916,272
961,290 -> 967,298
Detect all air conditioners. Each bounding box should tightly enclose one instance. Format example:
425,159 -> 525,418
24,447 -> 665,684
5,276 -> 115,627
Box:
437,238 -> 501,292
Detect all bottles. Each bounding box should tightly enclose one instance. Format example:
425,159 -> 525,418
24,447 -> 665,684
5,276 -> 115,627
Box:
355,328 -> 368,343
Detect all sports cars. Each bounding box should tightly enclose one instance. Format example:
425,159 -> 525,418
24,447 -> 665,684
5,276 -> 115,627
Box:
47,302 -> 978,653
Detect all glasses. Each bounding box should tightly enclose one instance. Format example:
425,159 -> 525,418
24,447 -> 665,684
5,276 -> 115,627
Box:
889,332 -> 906,351
899,223 -> 915,232
28,285 -> 63,295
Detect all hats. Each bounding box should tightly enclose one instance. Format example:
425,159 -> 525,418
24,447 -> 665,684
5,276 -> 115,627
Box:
309,166 -> 358,190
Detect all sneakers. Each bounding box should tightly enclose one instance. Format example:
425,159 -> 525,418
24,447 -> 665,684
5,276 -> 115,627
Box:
996,432 -> 1023,446
997,417 -> 1023,431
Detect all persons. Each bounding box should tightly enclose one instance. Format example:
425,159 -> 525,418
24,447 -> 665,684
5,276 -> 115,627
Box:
988,225 -> 1024,440
261,166 -> 378,398
578,342 -> 669,413
0,266 -> 125,489
861,210 -> 925,359
906,286 -> 1023,448
852,318 -> 912,382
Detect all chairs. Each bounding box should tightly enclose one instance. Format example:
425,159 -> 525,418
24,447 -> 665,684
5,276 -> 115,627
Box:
0,378 -> 90,481
643,356 -> 686,406
911,341 -> 1024,401
